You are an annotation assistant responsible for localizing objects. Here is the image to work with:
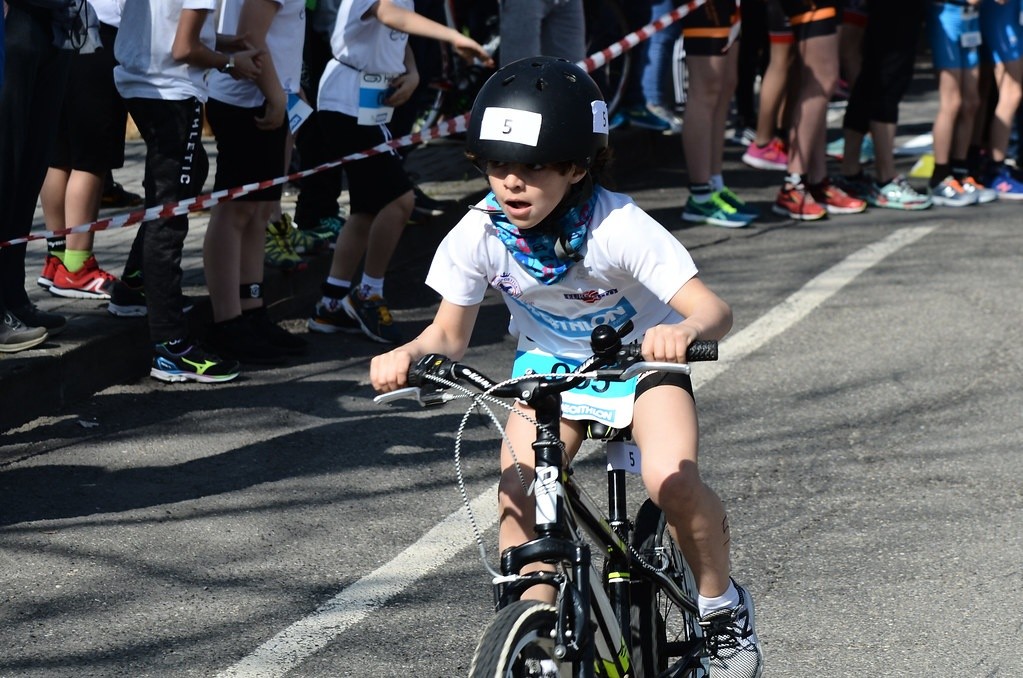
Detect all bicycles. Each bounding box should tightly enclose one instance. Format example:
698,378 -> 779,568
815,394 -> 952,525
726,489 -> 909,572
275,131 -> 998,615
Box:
373,318 -> 720,678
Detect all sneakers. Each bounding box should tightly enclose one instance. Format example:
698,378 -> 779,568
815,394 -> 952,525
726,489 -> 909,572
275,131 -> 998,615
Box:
608,111 -> 623,127
681,160 -> 1023,229
262,185 -> 449,273
622,106 -> 671,131
107,279 -> 194,317
742,136 -> 791,170
15,299 -> 67,334
309,301 -> 361,334
342,287 -> 403,343
0,306 -> 48,352
150,339 -> 242,382
699,575 -> 764,678
49,254 -> 117,299
37,253 -> 62,288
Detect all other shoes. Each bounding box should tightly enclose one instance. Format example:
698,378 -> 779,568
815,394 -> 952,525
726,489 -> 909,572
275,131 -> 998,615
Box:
97,182 -> 141,208
249,313 -> 309,353
204,320 -> 284,363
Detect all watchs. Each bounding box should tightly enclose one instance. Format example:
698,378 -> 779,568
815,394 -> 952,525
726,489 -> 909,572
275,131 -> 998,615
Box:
221,55 -> 234,74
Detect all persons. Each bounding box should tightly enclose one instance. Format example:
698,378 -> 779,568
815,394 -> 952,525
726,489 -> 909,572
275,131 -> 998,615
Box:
583,0 -> 1023,227
0,0 -> 586,383
370,56 -> 764,678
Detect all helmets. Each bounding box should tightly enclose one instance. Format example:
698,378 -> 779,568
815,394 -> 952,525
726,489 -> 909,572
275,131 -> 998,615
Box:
470,55 -> 609,163
